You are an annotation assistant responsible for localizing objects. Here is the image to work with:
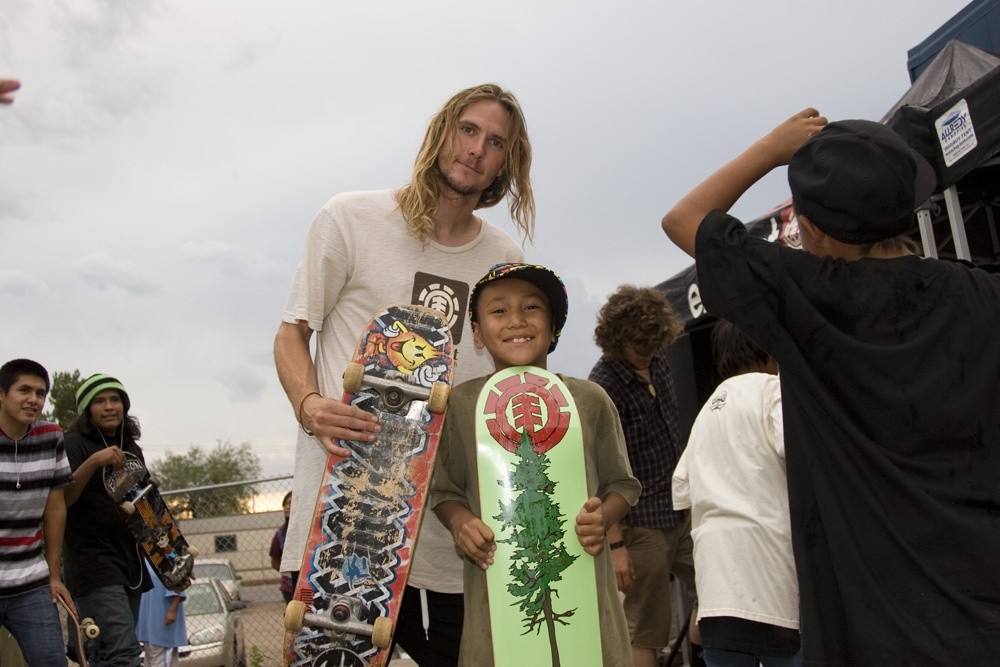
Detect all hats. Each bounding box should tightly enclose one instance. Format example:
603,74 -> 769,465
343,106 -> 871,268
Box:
74,372 -> 131,416
469,262 -> 568,354
788,119 -> 938,244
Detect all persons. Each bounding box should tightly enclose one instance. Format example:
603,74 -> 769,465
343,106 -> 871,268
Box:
61,372 -> 151,667
585,280 -> 796,667
662,108 -> 1000,667
0,359 -> 75,667
137,550 -> 189,667
270,84 -> 526,667
430,262 -> 642,667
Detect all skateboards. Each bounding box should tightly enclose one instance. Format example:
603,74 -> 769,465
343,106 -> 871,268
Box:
280,304 -> 457,667
53,591 -> 100,667
101,452 -> 196,592
472,366 -> 606,667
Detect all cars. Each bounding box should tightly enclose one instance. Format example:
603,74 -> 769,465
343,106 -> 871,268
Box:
192,559 -> 243,602
167,577 -> 244,664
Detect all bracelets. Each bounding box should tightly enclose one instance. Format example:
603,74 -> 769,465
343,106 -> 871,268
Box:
610,540 -> 626,550
299,392 -> 322,436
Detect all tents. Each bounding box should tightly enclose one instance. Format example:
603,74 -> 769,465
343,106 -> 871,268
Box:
873,38 -> 1000,262
617,194 -> 809,322
